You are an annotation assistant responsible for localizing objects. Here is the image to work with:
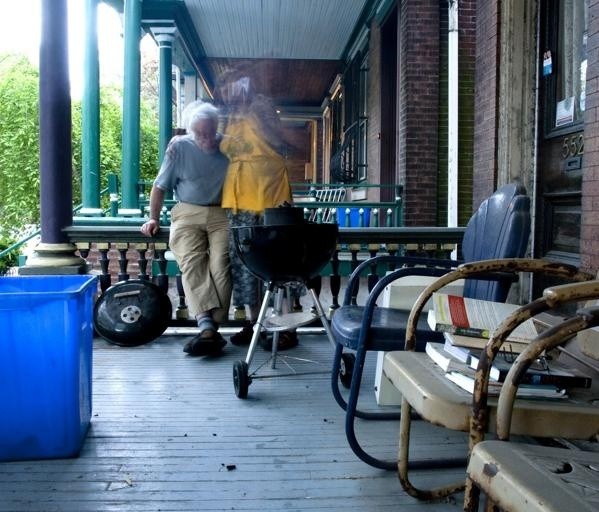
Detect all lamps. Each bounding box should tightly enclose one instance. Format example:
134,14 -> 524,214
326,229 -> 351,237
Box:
304,162 -> 313,183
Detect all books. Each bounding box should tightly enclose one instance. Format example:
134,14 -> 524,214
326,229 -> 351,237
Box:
420,291 -> 592,402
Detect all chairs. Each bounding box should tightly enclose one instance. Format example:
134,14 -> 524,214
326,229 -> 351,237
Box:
464,307 -> 599,512
332,185 -> 531,470
385,258 -> 599,499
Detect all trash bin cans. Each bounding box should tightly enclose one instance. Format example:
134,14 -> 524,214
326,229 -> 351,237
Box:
0,274 -> 99,462
336,206 -> 371,249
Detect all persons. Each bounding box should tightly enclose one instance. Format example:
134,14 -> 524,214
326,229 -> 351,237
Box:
136,102 -> 234,359
165,67 -> 308,353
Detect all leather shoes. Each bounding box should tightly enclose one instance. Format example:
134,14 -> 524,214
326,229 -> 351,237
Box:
183,333 -> 201,352
230,320 -> 267,346
190,332 -> 228,355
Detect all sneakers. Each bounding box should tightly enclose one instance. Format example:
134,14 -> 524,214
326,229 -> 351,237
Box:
260,331 -> 299,351
